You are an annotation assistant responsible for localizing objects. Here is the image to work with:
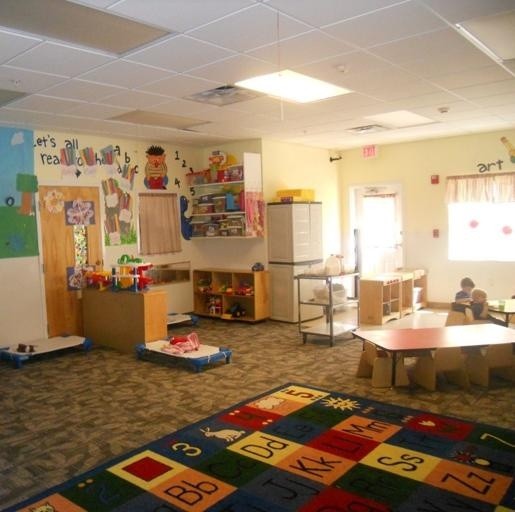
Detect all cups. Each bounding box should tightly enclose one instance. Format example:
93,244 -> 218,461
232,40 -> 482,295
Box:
498,300 -> 506,311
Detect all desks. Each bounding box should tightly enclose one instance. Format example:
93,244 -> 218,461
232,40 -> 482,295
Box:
347,322 -> 514,393
462,296 -> 514,324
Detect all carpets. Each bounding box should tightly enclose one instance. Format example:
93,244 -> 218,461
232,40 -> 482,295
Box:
3,381 -> 514,512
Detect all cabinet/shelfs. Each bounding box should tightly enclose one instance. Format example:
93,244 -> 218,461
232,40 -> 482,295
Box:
192,267 -> 272,323
358,268 -> 429,327
266,200 -> 325,324
294,269 -> 359,346
184,152 -> 262,240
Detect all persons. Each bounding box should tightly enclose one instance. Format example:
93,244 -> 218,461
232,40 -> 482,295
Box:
456,277 -> 475,306
469,290 -> 505,326
